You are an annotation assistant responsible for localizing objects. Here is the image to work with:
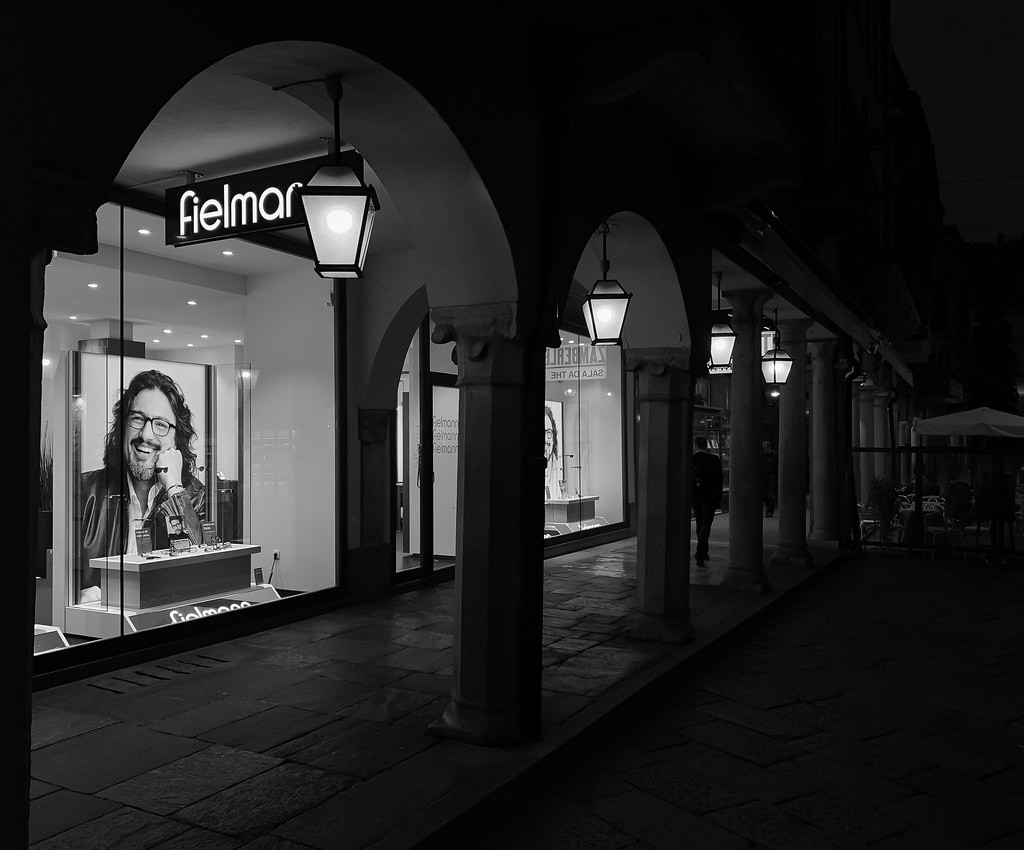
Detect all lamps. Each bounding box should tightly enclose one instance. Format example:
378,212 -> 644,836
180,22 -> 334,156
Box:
760,309 -> 795,384
296,75 -> 380,277
707,270 -> 740,368
582,221 -> 634,346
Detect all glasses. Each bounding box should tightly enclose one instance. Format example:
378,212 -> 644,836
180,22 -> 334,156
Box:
126,410 -> 177,437
545,428 -> 554,440
141,536 -> 231,557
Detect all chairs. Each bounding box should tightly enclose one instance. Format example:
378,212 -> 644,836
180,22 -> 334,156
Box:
856,484 -> 1024,552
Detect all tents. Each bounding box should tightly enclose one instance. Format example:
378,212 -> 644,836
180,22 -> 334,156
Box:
912,406 -> 1024,547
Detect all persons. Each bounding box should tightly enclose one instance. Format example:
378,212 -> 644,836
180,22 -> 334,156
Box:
687,435 -> 725,568
167,516 -> 188,547
759,440 -> 778,517
75,370 -> 209,607
543,405 -> 564,501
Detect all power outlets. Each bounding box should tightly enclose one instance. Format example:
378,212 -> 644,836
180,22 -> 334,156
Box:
273,550 -> 280,559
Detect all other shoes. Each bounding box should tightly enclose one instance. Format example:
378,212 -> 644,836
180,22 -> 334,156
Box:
765,511 -> 774,517
703,554 -> 709,561
694,553 -> 704,568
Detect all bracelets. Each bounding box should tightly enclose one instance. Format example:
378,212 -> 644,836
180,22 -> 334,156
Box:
166,484 -> 184,492
